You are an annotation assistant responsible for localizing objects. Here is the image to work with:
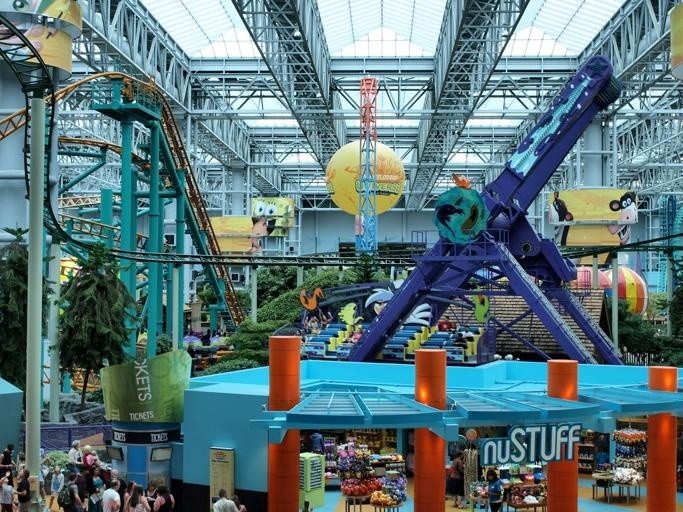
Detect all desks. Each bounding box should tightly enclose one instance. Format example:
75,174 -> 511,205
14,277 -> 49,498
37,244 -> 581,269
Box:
591,473 -> 645,505
504,496 -> 548,512
342,492 -> 406,512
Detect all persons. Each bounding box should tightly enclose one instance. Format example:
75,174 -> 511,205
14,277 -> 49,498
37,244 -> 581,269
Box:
345,325 -> 363,344
443,316 -> 472,348
295,311 -> 334,345
212,487 -> 246,512
449,450 -> 467,509
481,469 -> 504,512
0,439 -> 176,512
183,328 -> 226,370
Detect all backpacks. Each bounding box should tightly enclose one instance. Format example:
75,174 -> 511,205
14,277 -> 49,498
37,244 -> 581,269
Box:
57,483 -> 77,508
